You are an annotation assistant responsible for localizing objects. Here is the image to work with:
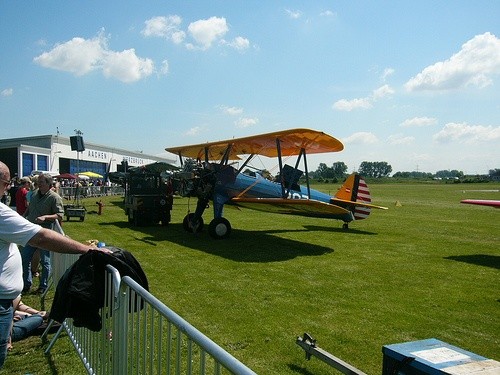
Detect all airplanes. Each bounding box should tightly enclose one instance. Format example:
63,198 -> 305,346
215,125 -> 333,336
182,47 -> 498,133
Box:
160,127 -> 389,240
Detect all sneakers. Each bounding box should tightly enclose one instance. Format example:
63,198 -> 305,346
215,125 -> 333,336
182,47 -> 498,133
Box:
33,272 -> 39,276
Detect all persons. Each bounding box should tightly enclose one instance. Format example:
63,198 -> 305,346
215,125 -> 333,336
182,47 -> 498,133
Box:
0,160 -> 114,371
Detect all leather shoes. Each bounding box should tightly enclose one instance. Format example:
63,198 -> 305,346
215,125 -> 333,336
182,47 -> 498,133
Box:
31,288 -> 47,295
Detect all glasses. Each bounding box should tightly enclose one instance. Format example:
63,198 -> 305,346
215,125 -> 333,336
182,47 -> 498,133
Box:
0,179 -> 12,186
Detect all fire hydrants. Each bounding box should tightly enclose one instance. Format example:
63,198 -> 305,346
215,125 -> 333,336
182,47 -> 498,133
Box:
96,199 -> 105,216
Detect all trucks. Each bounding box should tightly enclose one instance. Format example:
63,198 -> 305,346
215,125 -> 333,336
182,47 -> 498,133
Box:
123,173 -> 173,226
